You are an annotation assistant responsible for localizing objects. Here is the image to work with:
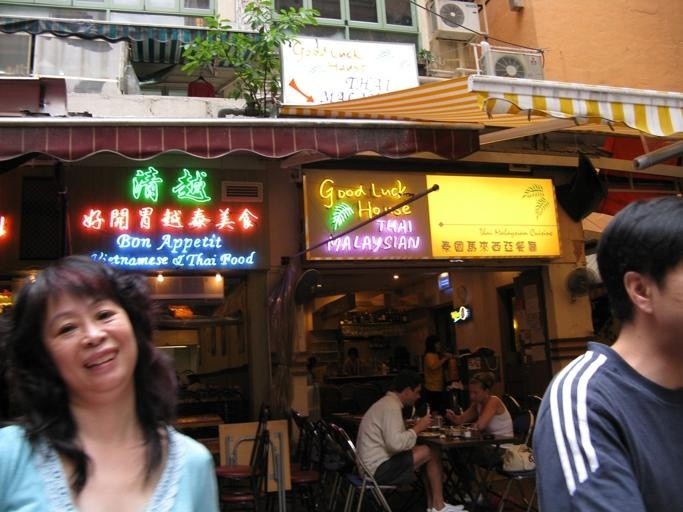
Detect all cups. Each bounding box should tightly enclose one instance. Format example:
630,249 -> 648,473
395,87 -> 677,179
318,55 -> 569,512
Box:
434,414 -> 443,428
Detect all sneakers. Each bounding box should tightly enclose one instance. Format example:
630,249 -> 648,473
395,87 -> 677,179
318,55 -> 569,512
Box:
426,503 -> 464,512
431,504 -> 467,512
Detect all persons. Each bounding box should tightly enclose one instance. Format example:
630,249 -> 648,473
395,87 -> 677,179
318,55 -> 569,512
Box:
357,367 -> 467,511
0,255 -> 220,512
342,346 -> 366,376
446,369 -> 515,503
532,196 -> 682,512
422,335 -> 452,415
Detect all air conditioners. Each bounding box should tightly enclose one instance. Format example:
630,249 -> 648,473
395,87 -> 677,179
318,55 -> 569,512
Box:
479,50 -> 545,81
429,0 -> 480,43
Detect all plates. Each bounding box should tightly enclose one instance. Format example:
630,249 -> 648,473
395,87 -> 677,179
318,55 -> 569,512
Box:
418,431 -> 440,438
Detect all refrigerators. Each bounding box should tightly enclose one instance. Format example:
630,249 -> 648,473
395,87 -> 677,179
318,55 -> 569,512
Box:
306,328 -> 344,376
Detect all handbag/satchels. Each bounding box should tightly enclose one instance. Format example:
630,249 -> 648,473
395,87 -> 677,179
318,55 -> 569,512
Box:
492,439 -> 537,473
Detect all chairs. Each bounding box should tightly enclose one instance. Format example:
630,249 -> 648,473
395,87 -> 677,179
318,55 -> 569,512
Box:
290,393 -> 543,512
210,409 -> 271,511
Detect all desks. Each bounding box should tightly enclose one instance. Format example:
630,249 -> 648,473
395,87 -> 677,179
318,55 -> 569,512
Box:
218,419 -> 292,511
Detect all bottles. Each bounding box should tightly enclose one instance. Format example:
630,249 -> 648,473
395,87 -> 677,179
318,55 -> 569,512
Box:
443,358 -> 466,427
463,423 -> 480,439
359,307 -> 408,323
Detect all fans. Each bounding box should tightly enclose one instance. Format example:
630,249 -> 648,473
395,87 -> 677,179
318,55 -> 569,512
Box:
564,267 -> 599,303
